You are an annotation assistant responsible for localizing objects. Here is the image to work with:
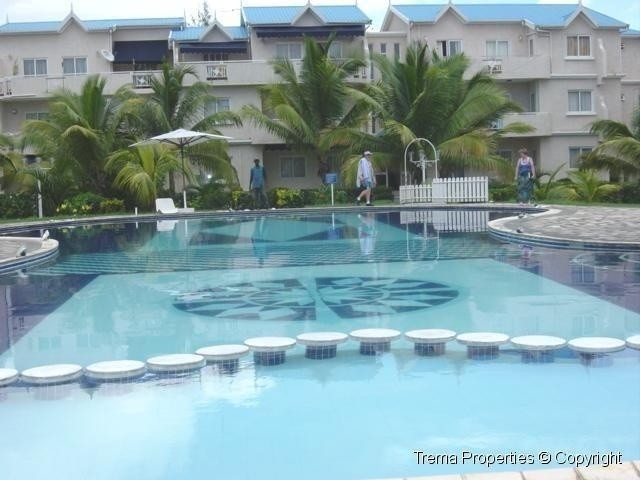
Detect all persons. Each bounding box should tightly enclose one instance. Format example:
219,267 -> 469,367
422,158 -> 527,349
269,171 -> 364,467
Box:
355,149 -> 377,206
355,212 -> 377,263
247,156 -> 276,211
250,218 -> 272,266
513,146 -> 539,208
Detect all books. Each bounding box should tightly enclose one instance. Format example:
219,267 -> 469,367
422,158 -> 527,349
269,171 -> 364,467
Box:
528,171 -> 533,179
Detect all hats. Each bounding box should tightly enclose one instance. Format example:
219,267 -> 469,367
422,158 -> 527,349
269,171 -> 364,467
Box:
364,151 -> 373,156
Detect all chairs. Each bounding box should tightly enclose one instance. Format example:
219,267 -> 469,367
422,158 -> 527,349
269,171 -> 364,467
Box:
156,220 -> 178,232
155,198 -> 179,214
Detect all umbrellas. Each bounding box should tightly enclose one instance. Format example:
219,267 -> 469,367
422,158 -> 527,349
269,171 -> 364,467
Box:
128,125 -> 235,208
148,220 -> 240,298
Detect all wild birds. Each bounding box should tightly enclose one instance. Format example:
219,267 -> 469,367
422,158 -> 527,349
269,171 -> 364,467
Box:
42,230 -> 49,241
517,213 -> 525,218
516,227 -> 522,233
16,247 -> 26,258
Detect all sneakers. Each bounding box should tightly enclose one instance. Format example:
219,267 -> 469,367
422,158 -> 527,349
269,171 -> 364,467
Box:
355,198 -> 374,206
